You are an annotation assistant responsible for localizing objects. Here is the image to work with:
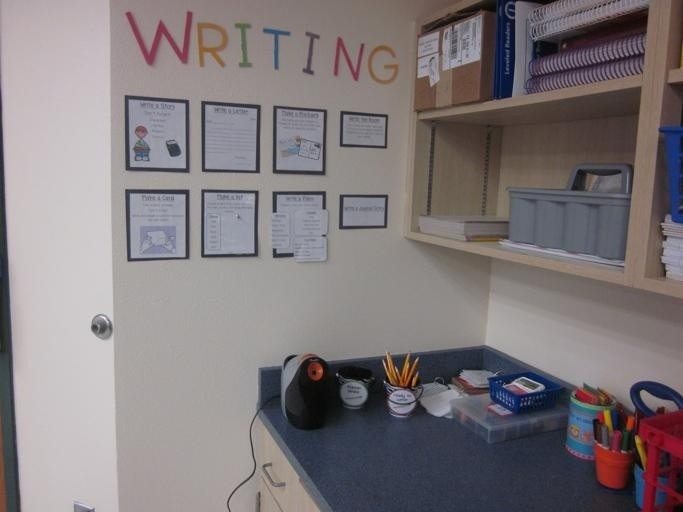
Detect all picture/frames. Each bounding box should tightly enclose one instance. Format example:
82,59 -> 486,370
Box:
340,110 -> 388,148
201,101 -> 261,174
272,191 -> 327,258
201,189 -> 258,257
339,195 -> 388,229
124,95 -> 190,173
272,105 -> 327,175
125,189 -> 189,261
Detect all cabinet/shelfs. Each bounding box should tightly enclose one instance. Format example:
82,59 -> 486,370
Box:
403,0 -> 659,292
633,0 -> 683,299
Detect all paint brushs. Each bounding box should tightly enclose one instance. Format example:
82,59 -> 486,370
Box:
592,408 -> 637,452
634,436 -> 648,470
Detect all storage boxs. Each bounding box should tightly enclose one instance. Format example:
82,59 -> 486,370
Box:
412,9 -> 496,112
450,393 -> 570,446
505,161 -> 631,263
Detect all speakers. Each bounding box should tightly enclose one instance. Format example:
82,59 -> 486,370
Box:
280,354 -> 332,430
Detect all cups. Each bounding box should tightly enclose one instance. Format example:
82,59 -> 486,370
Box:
335,366 -> 375,409
383,376 -> 421,417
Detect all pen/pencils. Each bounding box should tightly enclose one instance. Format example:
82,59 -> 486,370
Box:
381,347 -> 420,387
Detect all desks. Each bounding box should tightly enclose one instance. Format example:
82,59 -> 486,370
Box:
257,344 -> 637,511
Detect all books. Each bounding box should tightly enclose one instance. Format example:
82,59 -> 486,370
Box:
660,213 -> 683,282
417,214 -> 510,242
524,1 -> 651,94
